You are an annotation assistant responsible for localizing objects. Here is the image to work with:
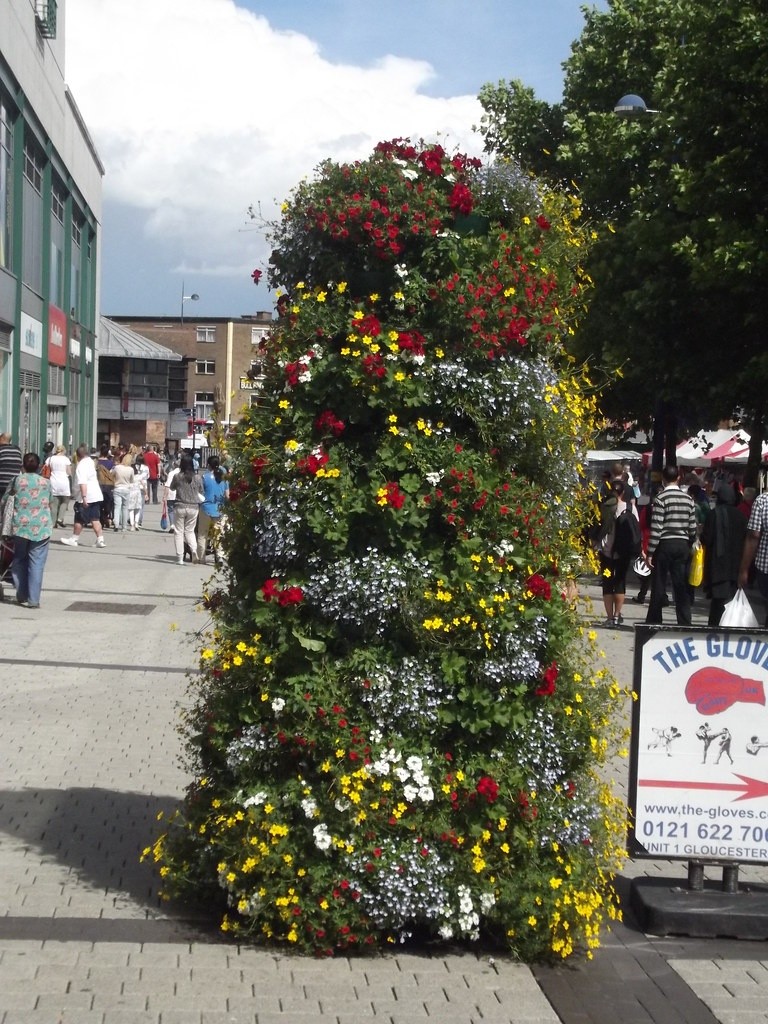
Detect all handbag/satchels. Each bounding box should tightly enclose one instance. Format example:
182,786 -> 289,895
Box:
688,536 -> 702,587
2,475 -> 18,539
42,457 -> 51,478
160,500 -> 168,529
197,492 -> 204,504
719,588 -> 759,628
95,464 -> 114,488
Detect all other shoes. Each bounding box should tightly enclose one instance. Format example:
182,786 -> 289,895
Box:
130,527 -> 134,531
114,525 -> 119,532
178,560 -> 183,564
56,520 -> 67,527
122,529 -> 127,531
192,551 -> 199,564
613,615 -> 624,625
135,525 -> 139,531
602,619 -> 615,628
169,527 -> 175,533
632,597 -> 644,604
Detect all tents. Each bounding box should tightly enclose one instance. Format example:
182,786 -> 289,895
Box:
642,429 -> 768,505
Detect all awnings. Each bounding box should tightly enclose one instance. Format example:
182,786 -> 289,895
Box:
544,448 -> 642,461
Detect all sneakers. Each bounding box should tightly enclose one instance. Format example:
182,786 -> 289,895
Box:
93,542 -> 106,548
61,537 -> 78,546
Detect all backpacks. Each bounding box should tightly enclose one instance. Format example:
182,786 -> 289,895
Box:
612,502 -> 643,561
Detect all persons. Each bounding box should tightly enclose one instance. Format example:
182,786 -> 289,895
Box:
585,464 -> 642,628
60,447 -> 106,548
221,450 -> 237,475
90,441 -> 161,532
39,442 -> 72,529
0,453 -> 53,608
0,433 -> 23,502
632,465 -> 711,625
70,443 -> 87,500
161,448 -> 203,565
700,471 -> 768,626
197,456 -> 230,567
